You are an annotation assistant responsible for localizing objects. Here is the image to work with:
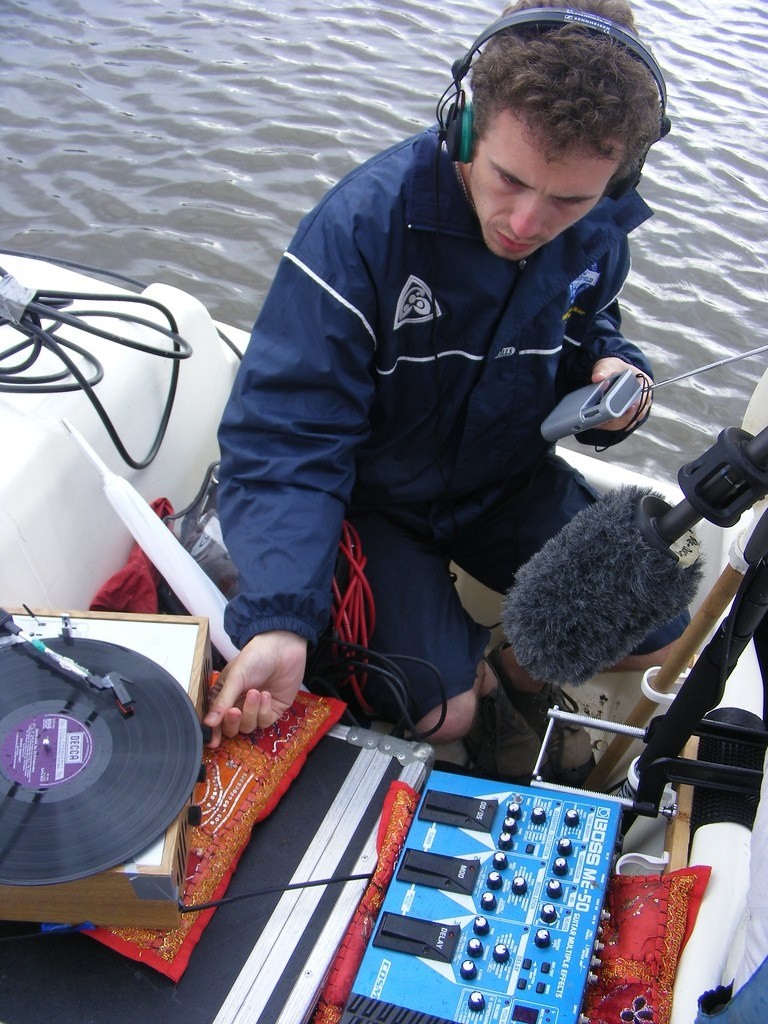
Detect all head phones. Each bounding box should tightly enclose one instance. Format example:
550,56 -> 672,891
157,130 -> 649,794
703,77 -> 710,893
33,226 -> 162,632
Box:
441,7 -> 671,201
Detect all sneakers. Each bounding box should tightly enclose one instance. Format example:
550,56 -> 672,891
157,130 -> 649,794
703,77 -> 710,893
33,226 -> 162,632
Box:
487,640 -> 596,779
461,656 -> 550,785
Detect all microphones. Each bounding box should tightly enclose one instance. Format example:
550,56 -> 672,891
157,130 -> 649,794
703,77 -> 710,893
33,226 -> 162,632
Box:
502,426 -> 768,686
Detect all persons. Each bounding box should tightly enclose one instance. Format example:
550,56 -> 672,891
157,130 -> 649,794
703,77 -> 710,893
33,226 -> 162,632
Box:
203,0 -> 690,747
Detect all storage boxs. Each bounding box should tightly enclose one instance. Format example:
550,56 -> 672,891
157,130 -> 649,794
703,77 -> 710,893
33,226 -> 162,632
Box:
0,715 -> 435,1024
